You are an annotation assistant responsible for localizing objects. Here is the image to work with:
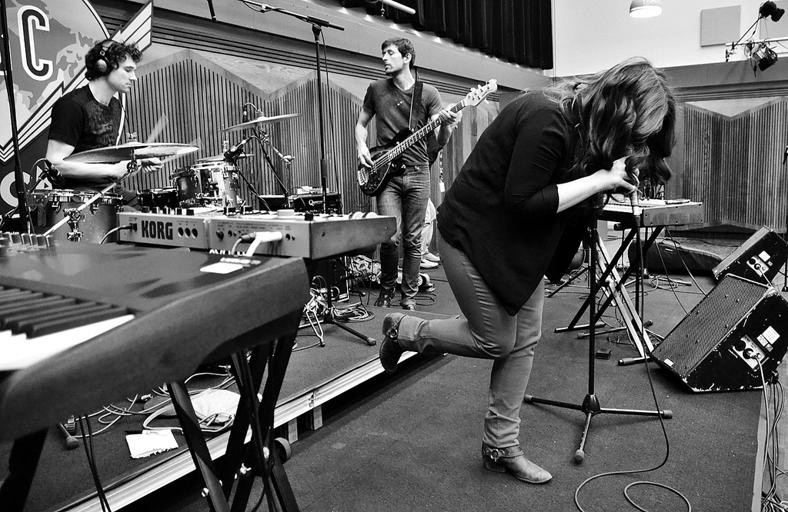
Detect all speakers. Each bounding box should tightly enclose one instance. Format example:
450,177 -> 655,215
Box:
646,273 -> 787,393
713,225 -> 787,284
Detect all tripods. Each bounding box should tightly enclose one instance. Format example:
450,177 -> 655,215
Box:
299,158 -> 378,346
524,236 -> 673,465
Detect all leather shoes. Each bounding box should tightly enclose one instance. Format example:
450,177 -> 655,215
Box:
480,442 -> 554,484
403,305 -> 417,311
374,287 -> 396,308
379,312 -> 406,379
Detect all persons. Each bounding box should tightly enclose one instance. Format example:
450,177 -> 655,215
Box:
45,38 -> 141,183
355,37 -> 462,311
380,57 -> 677,486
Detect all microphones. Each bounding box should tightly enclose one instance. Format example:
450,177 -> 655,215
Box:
224,135 -> 256,160
630,174 -> 640,210
242,105 -> 249,121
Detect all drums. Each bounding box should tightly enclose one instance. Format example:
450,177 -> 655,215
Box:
169,165 -> 221,209
136,187 -> 179,207
196,165 -> 242,209
33,187 -> 119,244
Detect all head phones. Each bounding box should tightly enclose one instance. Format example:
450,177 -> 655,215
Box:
95,41 -> 110,75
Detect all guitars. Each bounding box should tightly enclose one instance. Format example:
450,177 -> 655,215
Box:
356,79 -> 498,196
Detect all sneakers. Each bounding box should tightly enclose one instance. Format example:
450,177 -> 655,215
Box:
420,258 -> 439,270
424,252 -> 442,263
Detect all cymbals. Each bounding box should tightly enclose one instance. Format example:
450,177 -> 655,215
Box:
197,153 -> 256,162
218,114 -> 298,132
63,142 -> 199,163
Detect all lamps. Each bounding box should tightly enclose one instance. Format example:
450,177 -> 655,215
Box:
728,0 -> 787,79
625,2 -> 664,22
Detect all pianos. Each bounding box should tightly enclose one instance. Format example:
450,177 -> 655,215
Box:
602,192 -> 706,226
